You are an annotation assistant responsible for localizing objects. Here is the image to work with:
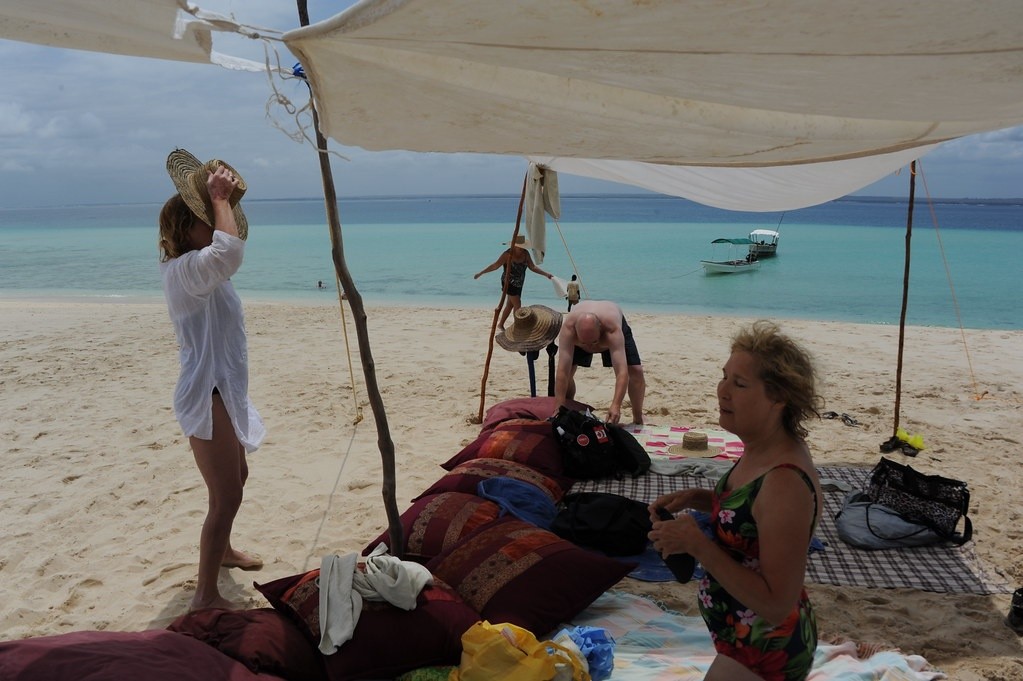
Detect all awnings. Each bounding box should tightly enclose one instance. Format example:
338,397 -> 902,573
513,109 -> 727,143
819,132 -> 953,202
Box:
1,0 -> 1023,535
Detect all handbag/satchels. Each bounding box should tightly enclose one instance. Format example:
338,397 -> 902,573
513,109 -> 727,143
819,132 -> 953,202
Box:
834,491 -> 946,550
868,456 -> 973,548
458,620 -> 616,681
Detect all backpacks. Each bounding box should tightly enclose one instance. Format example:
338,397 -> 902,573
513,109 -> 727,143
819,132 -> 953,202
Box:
551,404 -> 651,480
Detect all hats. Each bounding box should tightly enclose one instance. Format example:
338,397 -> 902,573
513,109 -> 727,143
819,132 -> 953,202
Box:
166,148 -> 248,241
501,234 -> 535,249
495,304 -> 563,351
668,431 -> 722,458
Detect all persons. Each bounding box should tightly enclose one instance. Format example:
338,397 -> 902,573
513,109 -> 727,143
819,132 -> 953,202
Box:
159,148 -> 264,610
553,302 -> 646,426
566,274 -> 580,312
474,234 -> 553,332
649,330 -> 823,681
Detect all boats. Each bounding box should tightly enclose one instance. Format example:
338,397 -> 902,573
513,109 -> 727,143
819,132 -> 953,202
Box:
748,229 -> 779,258
701,238 -> 761,276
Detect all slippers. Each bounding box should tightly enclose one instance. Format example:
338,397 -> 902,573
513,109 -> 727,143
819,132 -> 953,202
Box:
822,411 -> 838,419
842,413 -> 855,427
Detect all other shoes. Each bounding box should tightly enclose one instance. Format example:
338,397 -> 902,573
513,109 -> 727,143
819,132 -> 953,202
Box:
902,442 -> 918,457
880,436 -> 903,450
